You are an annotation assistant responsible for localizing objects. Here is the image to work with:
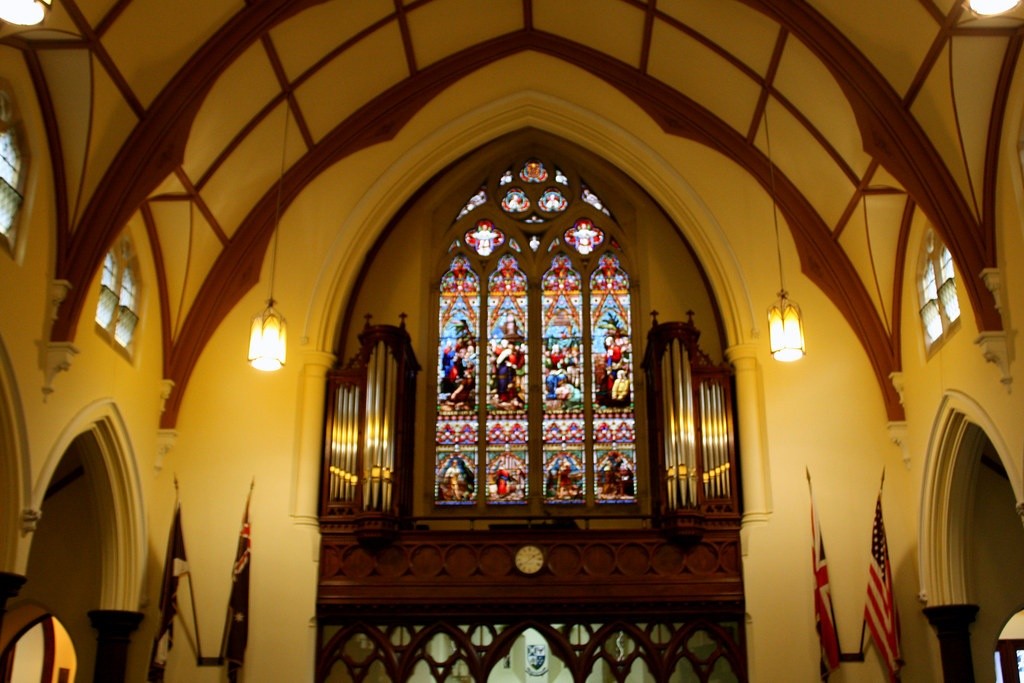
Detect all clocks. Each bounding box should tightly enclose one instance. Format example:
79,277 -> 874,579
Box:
516,545 -> 544,574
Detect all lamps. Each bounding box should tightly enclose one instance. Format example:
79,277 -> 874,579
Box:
248,85 -> 286,371
763,75 -> 806,362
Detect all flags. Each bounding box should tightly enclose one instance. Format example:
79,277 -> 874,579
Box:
864,495 -> 907,683
155,510 -> 187,665
809,498 -> 842,683
219,508 -> 251,683
